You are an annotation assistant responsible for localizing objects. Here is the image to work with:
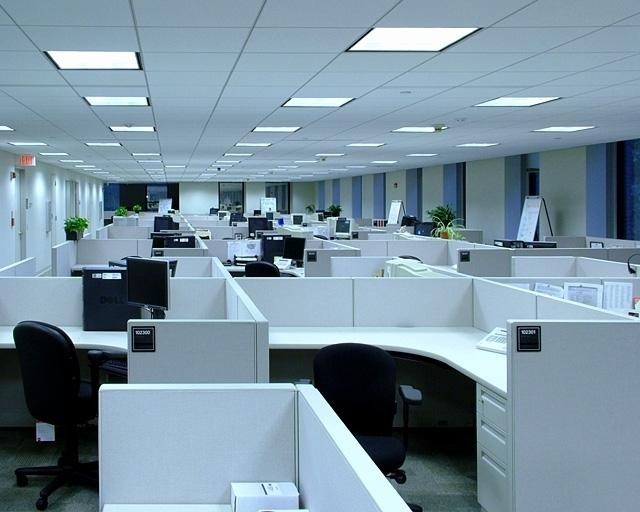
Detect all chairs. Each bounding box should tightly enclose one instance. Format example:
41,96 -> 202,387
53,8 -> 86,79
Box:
398,256 -> 423,264
312,235 -> 328,240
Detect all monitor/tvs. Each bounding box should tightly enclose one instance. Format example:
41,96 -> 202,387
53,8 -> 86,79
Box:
333,219 -> 352,240
313,214 -> 322,221
253,209 -> 262,216
167,209 -> 175,215
248,217 -> 274,239
292,214 -> 304,225
210,208 -> 219,214
285,237 -> 306,266
316,209 -> 325,212
169,259 -> 178,278
224,205 -> 231,210
154,216 -> 172,232
267,213 -> 273,220
229,212 -> 241,225
383,258 -> 451,278
163,215 -> 170,217
167,236 -> 196,248
218,210 -> 227,220
325,211 -> 332,217
126,257 -> 170,319
414,221 -> 436,237
236,208 -> 241,211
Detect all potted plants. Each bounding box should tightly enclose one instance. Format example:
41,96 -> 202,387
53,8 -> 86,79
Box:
431,216 -> 465,238
63,216 -> 90,240
133,205 -> 142,214
327,205 -> 343,217
427,200 -> 457,237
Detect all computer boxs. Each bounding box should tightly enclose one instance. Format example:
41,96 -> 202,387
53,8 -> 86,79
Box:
494,238 -> 525,249
151,236 -> 165,247
151,232 -> 182,239
524,241 -> 557,248
260,235 -> 291,263
81,266 -> 127,332
108,259 -> 126,267
172,222 -> 179,230
